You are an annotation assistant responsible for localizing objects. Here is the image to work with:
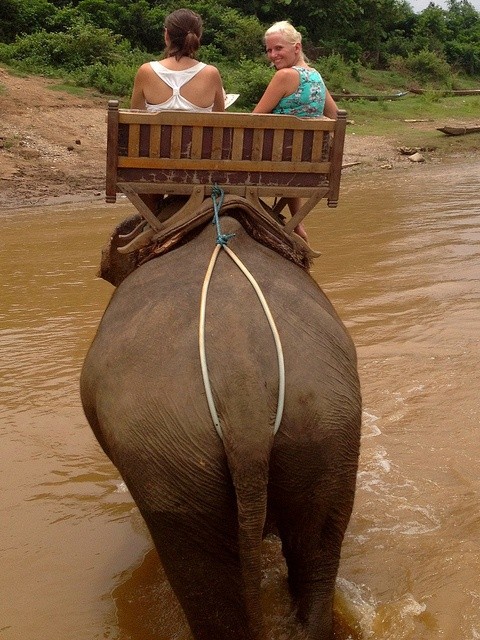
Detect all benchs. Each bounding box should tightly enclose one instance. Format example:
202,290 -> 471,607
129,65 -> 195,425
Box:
105,100 -> 349,258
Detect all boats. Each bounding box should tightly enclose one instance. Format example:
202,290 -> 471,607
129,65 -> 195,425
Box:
331,91 -> 410,101
435,125 -> 480,135
410,89 -> 480,96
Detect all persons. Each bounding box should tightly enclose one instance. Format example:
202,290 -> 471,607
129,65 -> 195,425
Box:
128,7 -> 226,220
252,18 -> 338,239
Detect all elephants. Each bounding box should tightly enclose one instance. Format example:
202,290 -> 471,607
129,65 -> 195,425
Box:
80,196 -> 363,640
